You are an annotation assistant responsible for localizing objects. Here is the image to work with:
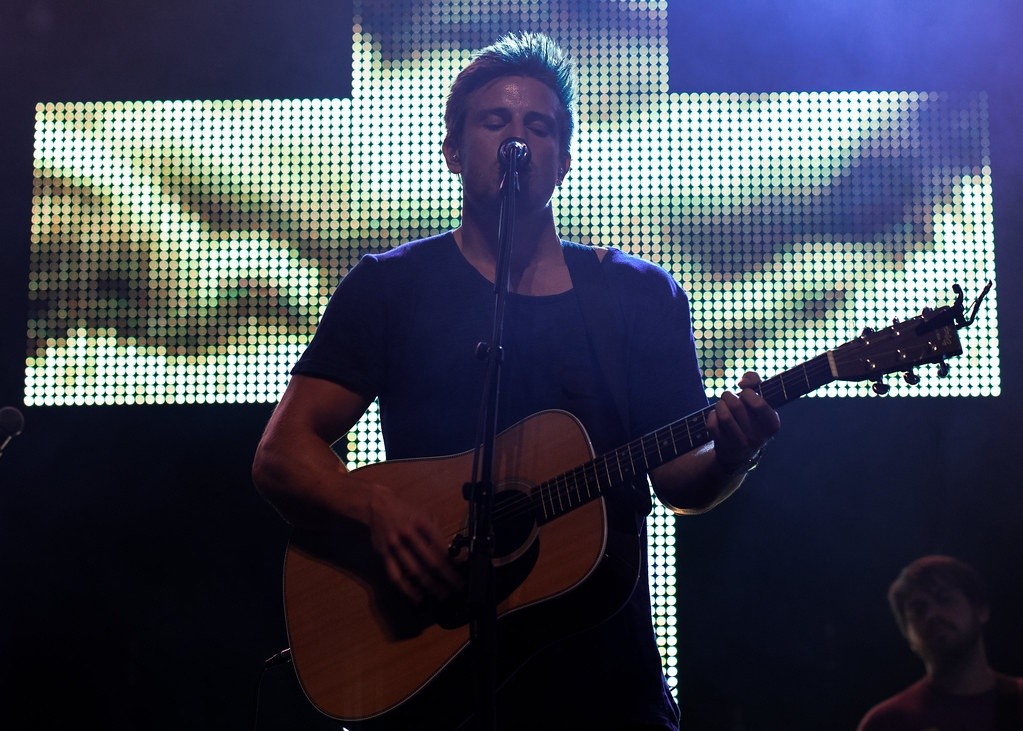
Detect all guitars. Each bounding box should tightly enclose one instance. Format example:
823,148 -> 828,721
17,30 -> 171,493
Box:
281,303 -> 963,731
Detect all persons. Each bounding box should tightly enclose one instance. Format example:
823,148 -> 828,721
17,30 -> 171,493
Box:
859,556 -> 1023,731
250,29 -> 779,731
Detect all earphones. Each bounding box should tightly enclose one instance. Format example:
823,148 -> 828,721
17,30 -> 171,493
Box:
558,167 -> 565,177
452,153 -> 459,163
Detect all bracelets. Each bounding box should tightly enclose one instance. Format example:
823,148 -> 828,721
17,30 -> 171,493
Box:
712,447 -> 763,472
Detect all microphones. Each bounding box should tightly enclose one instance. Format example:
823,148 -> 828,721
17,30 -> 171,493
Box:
0,406 -> 25,458
498,137 -> 531,168
